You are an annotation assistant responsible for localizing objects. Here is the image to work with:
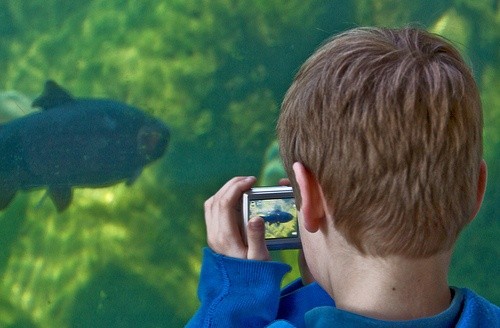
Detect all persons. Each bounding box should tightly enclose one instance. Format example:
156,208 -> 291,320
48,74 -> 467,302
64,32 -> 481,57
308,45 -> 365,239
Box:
181,21 -> 499,328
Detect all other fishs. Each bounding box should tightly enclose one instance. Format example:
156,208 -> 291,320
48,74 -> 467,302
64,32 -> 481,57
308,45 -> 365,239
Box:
0,80 -> 170,213
257,209 -> 293,226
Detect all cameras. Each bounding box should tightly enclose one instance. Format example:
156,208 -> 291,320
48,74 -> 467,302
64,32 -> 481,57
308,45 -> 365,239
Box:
239,187 -> 301,249
